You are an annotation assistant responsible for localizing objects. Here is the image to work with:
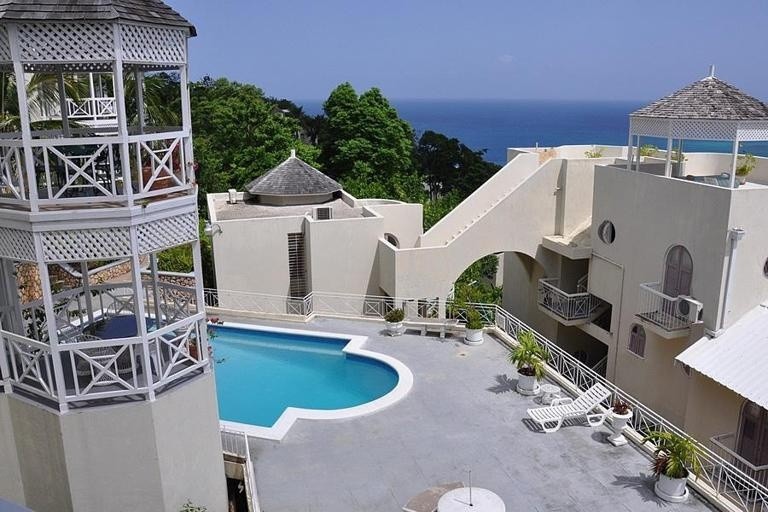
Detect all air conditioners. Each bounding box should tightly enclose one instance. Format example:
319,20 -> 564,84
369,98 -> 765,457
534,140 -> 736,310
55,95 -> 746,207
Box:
677,293 -> 705,324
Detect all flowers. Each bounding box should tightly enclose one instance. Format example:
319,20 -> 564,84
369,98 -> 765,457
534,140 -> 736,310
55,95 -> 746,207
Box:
206,316 -> 224,327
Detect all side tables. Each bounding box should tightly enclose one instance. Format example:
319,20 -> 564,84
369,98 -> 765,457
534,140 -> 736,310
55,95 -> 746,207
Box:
541,384 -> 561,404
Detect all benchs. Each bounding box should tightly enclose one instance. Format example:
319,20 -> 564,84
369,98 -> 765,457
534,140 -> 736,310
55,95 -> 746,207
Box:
403,482 -> 464,511
403,322 -> 465,341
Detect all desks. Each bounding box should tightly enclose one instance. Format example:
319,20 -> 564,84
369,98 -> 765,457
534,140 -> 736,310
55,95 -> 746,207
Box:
436,486 -> 507,512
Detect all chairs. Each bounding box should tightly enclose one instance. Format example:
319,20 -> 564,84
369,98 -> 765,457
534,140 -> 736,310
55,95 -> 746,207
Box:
64,333 -> 119,386
159,302 -> 197,341
526,382 -> 611,433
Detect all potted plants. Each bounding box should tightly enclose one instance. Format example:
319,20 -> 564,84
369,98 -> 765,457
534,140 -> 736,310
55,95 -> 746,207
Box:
384,308 -> 404,335
506,329 -> 551,395
463,309 -> 484,346
732,152 -> 760,183
607,393 -> 633,447
643,429 -> 701,503
